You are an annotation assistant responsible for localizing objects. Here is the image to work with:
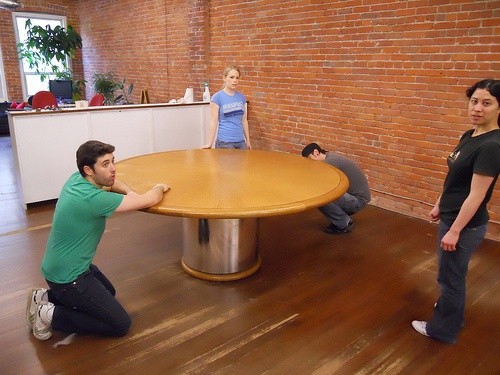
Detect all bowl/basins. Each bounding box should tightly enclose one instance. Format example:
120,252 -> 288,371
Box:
75,100 -> 88,108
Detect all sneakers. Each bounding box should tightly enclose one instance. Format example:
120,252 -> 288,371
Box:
25,287 -> 47,328
34,301 -> 53,340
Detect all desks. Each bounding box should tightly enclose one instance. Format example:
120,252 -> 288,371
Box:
103,149 -> 351,282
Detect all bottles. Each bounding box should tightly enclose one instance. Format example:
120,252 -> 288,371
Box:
203,84 -> 210,102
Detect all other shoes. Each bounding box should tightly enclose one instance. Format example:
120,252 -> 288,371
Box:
329,221 -> 352,226
412,320 -> 456,343
325,226 -> 350,234
433,303 -> 438,309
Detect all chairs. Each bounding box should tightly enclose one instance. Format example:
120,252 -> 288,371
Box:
89,94 -> 104,106
32,91 -> 57,108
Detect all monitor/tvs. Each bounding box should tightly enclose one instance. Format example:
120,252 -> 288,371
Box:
49,80 -> 73,104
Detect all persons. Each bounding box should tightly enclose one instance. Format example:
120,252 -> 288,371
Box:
24,140 -> 173,340
200,65 -> 253,151
301,143 -> 371,234
411,78 -> 500,344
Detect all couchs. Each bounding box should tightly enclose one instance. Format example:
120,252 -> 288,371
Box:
0,101 -> 21,134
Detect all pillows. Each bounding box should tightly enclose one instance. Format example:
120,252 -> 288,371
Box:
7,101 -> 25,110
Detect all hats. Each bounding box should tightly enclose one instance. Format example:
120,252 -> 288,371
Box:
302,143 -> 318,155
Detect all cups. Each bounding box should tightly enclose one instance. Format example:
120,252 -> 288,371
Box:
186,88 -> 193,102
